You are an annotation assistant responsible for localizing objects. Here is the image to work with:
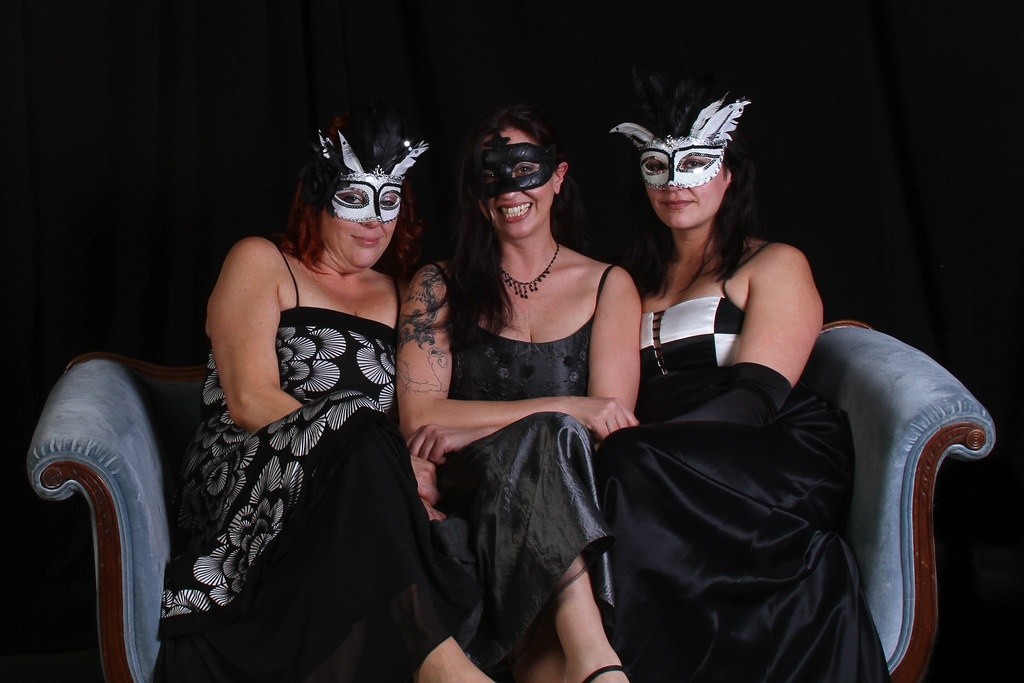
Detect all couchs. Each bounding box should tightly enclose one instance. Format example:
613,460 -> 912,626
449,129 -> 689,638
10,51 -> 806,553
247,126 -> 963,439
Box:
28,322 -> 994,682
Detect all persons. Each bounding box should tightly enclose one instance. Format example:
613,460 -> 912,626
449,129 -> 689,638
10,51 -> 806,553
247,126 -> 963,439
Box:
399,108 -> 648,683
156,109 -> 499,682
591,90 -> 892,683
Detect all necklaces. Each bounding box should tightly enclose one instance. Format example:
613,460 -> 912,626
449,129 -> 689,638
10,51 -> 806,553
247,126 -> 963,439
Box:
499,242 -> 559,301
